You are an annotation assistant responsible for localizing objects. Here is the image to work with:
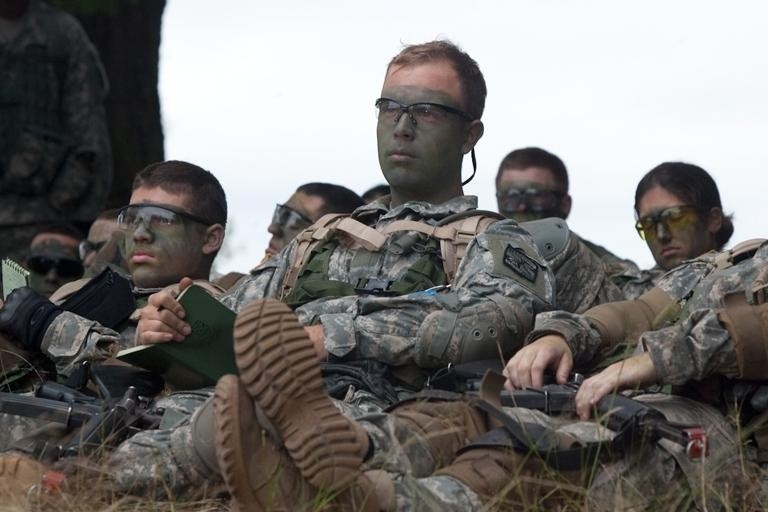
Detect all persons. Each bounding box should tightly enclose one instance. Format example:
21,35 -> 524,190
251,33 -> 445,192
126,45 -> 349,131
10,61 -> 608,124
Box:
1,2 -> 164,275
1,40 -> 768,512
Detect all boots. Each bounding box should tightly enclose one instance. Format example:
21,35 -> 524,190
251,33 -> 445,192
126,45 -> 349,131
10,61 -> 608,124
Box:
214,299 -> 395,512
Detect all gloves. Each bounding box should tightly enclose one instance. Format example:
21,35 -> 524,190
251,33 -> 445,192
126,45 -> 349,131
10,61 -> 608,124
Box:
0,288 -> 63,352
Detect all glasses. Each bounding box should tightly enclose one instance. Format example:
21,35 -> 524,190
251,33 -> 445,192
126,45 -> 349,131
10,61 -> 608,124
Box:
636,205 -> 707,240
29,257 -> 83,276
497,188 -> 566,212
114,204 -> 212,230
375,97 -> 472,123
273,204 -> 312,229
79,241 -> 122,261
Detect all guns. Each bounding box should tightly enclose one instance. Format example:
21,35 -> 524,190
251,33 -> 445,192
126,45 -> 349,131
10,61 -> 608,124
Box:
445,358 -> 710,458
0,359 -> 164,510
